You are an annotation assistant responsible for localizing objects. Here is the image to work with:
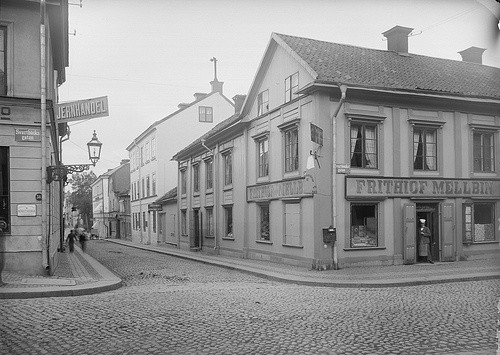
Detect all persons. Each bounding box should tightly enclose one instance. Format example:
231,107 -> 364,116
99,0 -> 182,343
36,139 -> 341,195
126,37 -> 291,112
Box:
65,230 -> 78,253
418,219 -> 434,264
78,232 -> 87,253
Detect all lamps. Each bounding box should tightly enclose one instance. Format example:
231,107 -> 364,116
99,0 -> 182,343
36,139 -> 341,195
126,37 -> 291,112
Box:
68,0 -> 82,7
46,130 -> 102,184
69,29 -> 77,35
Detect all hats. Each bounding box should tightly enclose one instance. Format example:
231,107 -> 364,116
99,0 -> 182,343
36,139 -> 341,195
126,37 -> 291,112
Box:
420,219 -> 426,223
